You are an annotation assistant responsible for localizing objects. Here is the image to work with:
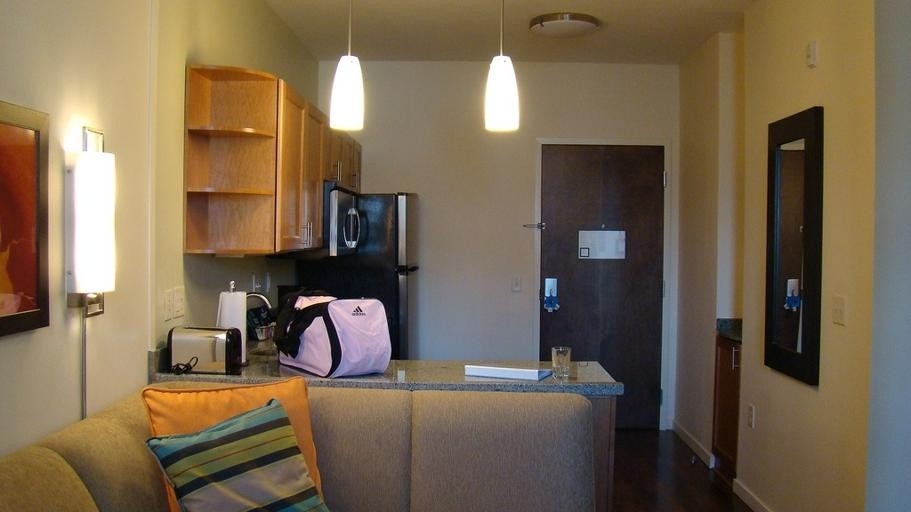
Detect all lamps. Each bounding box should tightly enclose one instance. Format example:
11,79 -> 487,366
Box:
526,12 -> 605,45
328,1 -> 523,135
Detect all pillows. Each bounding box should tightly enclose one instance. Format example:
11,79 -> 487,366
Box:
138,373 -> 329,511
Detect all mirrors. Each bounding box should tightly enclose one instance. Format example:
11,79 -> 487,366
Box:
762,104 -> 826,387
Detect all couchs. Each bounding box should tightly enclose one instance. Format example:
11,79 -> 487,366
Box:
1,379 -> 600,511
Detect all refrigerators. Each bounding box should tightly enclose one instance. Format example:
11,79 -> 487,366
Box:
300,194 -> 419,360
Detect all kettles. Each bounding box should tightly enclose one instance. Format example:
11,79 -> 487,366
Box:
216,280 -> 248,367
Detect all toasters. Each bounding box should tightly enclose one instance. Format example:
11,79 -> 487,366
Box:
169,325 -> 241,376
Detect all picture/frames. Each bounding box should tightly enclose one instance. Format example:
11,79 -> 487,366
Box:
0,99 -> 52,338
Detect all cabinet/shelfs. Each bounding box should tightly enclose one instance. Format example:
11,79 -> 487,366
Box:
183,61 -> 365,259
712,335 -> 741,468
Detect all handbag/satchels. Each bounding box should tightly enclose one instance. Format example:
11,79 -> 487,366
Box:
278,287 -> 392,378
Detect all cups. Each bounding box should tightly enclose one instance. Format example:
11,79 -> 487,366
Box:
551,346 -> 571,377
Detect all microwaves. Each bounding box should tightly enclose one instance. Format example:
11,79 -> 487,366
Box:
328,186 -> 362,256
